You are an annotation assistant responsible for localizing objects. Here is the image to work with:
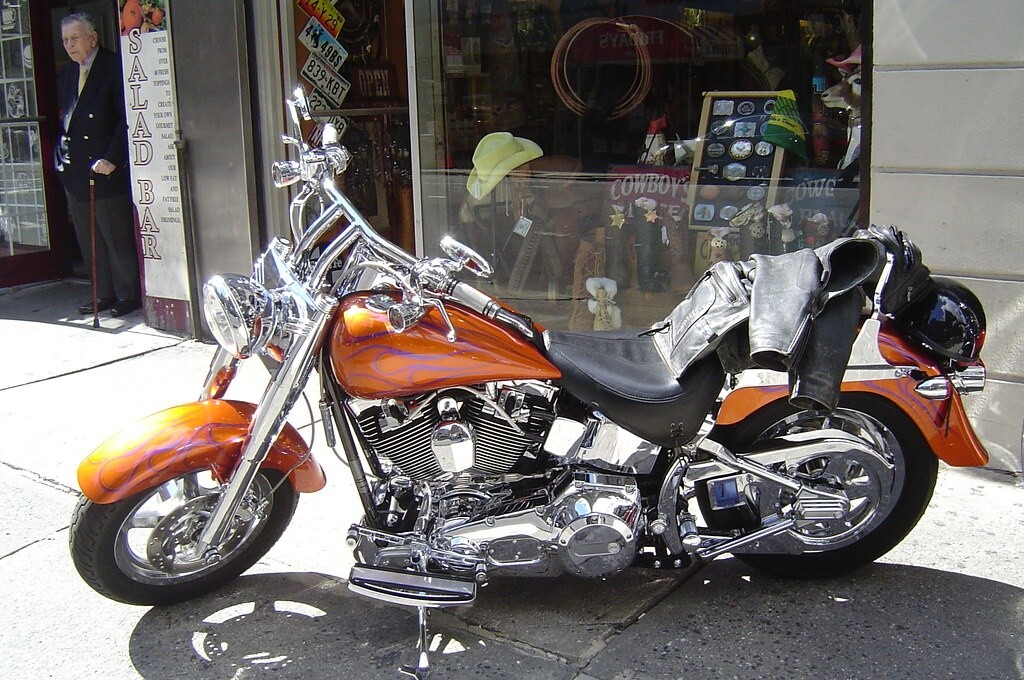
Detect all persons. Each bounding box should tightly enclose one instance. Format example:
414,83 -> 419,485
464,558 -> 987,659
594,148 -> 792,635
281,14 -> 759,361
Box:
50,12 -> 142,316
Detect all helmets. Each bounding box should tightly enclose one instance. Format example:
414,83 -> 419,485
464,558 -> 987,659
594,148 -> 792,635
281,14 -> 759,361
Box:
893,277 -> 986,363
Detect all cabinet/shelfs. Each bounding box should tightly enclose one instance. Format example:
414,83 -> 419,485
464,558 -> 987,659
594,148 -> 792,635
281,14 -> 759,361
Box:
438,0 -> 745,177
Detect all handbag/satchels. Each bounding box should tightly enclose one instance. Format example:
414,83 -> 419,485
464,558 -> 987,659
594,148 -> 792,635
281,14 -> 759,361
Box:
853,224 -> 930,315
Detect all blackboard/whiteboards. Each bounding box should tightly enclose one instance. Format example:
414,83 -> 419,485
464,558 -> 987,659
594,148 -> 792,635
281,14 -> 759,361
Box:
686,91 -> 794,230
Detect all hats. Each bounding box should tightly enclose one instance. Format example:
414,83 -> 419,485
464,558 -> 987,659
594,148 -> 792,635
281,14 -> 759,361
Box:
466,132 -> 543,201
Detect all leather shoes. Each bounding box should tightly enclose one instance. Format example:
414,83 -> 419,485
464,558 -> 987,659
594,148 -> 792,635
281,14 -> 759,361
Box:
111,301 -> 140,317
80,298 -> 115,314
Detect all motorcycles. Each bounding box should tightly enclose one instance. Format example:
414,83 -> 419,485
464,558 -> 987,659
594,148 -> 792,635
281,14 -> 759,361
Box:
65,89 -> 992,678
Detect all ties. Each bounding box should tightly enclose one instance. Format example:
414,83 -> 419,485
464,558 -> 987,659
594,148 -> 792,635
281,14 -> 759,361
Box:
78,68 -> 87,96
